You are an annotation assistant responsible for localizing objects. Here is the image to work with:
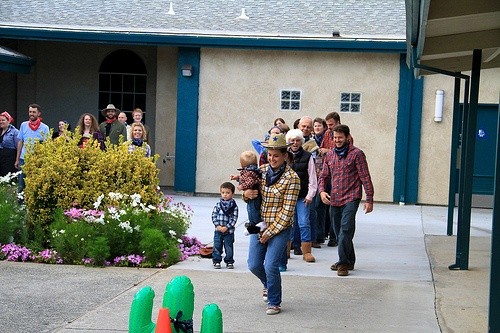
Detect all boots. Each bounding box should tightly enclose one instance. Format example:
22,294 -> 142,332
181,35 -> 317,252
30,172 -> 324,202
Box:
301,242 -> 315,262
287,241 -> 291,258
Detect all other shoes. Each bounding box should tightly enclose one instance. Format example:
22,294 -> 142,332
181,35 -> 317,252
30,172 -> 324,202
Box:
327,239 -> 337,247
266,305 -> 281,315
262,287 -> 270,303
313,242 -> 321,248
294,246 -> 303,255
226,262 -> 234,269
213,263 -> 221,269
330,263 -> 354,276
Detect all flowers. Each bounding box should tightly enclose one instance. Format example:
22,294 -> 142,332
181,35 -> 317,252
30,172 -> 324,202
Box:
0,123 -> 206,270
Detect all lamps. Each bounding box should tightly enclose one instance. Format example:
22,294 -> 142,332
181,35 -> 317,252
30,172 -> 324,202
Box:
180,64 -> 193,79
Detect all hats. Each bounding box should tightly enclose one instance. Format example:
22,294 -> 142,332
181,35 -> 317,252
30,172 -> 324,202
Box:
263,133 -> 294,149
101,104 -> 120,118
1,111 -> 14,123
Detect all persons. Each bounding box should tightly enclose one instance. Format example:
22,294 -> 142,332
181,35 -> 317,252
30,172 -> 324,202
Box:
259,111 -> 353,263
212,181 -> 239,269
0,112 -> 20,176
52,119 -> 73,142
15,103 -> 51,202
242,133 -> 301,314
75,103 -> 152,158
230,151 -> 268,237
316,125 -> 375,275
